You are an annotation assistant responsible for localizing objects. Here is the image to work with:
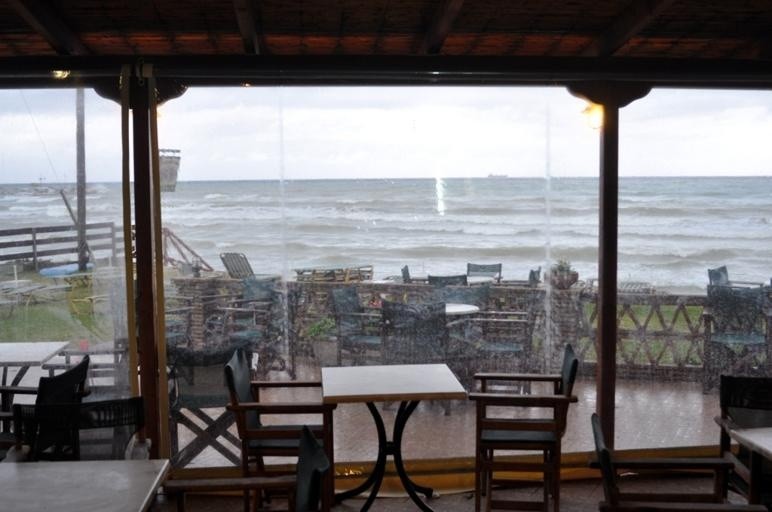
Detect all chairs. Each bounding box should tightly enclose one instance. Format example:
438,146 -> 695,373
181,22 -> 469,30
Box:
590,374 -> 772,512
11,354 -> 91,462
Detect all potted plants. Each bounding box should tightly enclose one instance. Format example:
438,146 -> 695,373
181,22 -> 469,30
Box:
545,257 -> 579,288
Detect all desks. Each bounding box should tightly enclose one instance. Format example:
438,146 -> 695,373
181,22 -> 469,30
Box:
0,341 -> 68,448
